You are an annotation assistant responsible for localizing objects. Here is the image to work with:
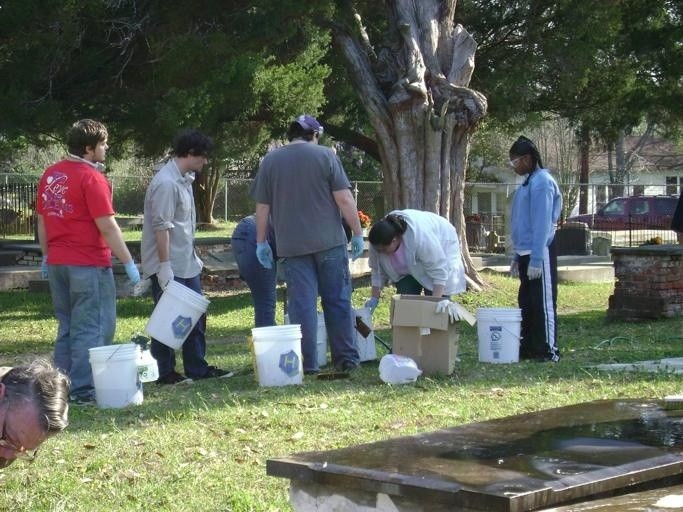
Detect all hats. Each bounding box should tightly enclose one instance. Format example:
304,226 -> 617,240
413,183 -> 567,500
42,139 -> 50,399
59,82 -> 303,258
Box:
291,114 -> 320,131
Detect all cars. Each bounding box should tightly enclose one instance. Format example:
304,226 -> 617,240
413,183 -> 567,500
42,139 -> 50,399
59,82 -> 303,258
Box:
464,211 -> 505,253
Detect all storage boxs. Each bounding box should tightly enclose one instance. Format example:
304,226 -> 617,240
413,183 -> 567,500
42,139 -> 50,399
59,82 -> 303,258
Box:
390,293 -> 477,375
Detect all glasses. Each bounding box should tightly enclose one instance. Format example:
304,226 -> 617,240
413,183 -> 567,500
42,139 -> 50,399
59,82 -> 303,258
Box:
508,155 -> 528,170
0,394 -> 40,464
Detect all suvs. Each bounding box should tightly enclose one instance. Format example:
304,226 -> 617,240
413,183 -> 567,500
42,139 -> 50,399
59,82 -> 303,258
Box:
560,194 -> 680,231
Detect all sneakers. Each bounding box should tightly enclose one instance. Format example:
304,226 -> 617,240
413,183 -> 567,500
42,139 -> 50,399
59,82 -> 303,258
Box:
154,370 -> 192,386
66,394 -> 96,408
303,369 -> 320,376
335,359 -> 359,374
183,365 -> 233,380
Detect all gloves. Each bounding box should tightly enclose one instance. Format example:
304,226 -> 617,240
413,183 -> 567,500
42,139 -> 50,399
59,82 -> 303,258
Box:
348,234 -> 365,262
433,297 -> 467,325
362,295 -> 380,316
40,255 -> 49,280
156,260 -> 175,292
508,260 -> 521,279
122,259 -> 140,283
254,239 -> 275,270
525,265 -> 543,281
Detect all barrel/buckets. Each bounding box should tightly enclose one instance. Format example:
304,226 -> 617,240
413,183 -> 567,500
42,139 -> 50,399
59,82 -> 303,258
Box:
251,324 -> 303,387
352,309 -> 377,364
477,308 -> 522,363
284,311 -> 328,367
87,343 -> 144,410
144,280 -> 211,350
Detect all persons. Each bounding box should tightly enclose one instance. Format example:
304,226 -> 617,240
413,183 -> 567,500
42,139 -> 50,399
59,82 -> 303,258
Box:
365,208 -> 466,315
0,354 -> 70,469
510,135 -> 560,363
247,115 -> 365,375
231,212 -> 352,328
140,130 -> 233,386
36,118 -> 140,406
